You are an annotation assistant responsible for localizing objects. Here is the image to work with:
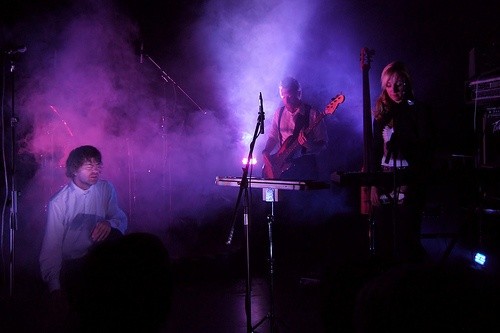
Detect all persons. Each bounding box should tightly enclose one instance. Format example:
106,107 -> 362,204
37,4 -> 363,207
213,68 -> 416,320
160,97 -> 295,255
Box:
260,76 -> 330,171
369,59 -> 422,209
37,145 -> 131,297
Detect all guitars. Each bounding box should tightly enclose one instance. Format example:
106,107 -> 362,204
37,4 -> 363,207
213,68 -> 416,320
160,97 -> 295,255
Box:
261,93 -> 345,179
359,48 -> 374,173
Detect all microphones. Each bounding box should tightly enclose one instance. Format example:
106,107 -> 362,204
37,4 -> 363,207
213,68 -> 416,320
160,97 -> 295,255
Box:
258,92 -> 264,134
384,124 -> 391,164
139,44 -> 144,63
5,45 -> 28,55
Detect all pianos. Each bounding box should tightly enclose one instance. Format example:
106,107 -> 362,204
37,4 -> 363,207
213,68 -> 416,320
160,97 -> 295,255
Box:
214,175 -> 331,191
